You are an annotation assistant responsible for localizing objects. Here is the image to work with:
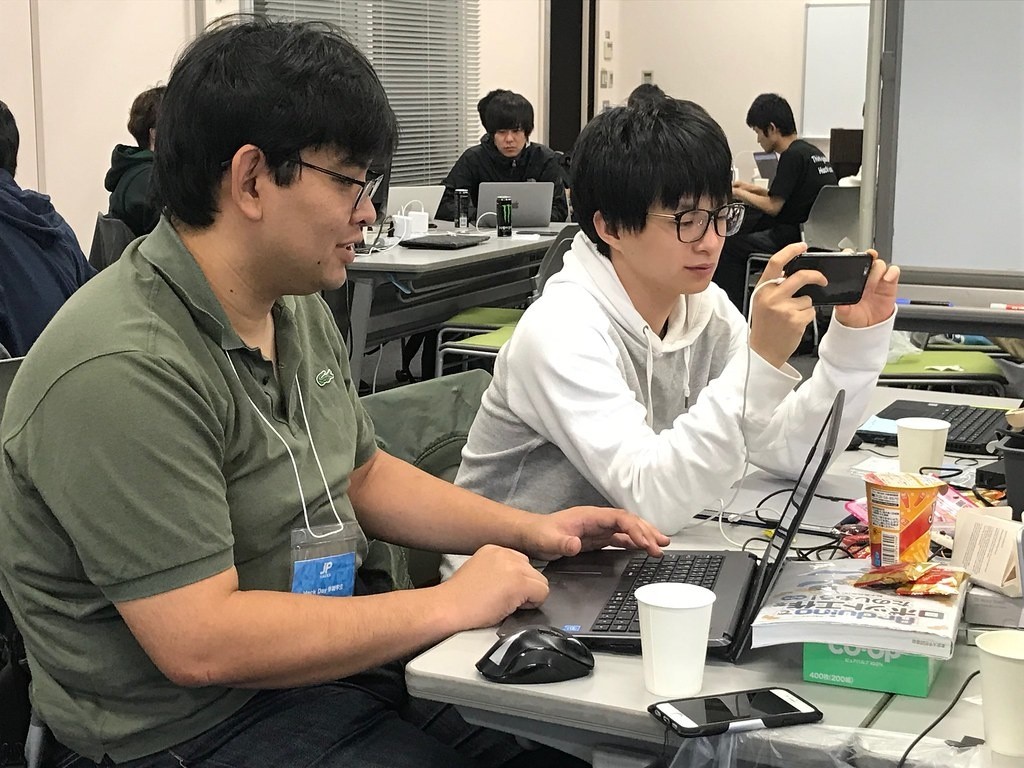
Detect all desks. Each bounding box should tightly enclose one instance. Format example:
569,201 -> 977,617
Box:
406,386 -> 1024,768
343,222 -> 576,396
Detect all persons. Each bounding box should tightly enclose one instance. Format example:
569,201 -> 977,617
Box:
727,94 -> 837,261
0,100 -> 98,360
0,14 -> 670,768
438,94 -> 900,584
434,89 -> 569,222
105,86 -> 168,236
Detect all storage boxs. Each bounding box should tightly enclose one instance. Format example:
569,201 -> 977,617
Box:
802,644 -> 945,698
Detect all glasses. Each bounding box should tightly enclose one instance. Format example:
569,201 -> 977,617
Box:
221,149 -> 384,210
646,199 -> 746,243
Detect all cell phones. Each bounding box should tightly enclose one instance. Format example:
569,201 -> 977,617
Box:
516,231 -> 559,236
648,687 -> 824,738
782,251 -> 873,305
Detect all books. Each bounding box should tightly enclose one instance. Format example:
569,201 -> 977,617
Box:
750,561 -> 969,661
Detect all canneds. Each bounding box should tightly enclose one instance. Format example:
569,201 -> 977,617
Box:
454,189 -> 469,228
496,196 -> 512,237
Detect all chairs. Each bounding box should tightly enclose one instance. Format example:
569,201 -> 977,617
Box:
436,238 -> 575,378
439,225 -> 578,342
741,186 -> 860,357
87,213 -> 136,269
361,369 -> 492,589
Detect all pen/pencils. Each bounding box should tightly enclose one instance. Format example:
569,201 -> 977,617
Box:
1006,305 -> 1024,310
910,300 -> 950,306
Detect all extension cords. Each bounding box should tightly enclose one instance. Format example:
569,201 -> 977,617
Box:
372,236 -> 403,249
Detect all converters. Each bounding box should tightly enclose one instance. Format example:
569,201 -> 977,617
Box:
976,458 -> 1006,489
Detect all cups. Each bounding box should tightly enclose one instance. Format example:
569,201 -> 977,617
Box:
633,581 -> 717,698
862,473 -> 946,567
896,417 -> 951,474
975,629 -> 1024,756
753,178 -> 769,189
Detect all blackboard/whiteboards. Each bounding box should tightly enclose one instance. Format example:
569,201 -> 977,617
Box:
801,4 -> 870,138
877,0 -> 1024,290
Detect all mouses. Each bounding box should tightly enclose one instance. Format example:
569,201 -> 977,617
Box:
845,434 -> 862,451
475,624 -> 595,685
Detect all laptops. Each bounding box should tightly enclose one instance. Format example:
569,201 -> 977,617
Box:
497,388 -> 846,664
471,182 -> 554,227
856,400 -> 1024,456
753,152 -> 779,189
384,186 -> 446,223
